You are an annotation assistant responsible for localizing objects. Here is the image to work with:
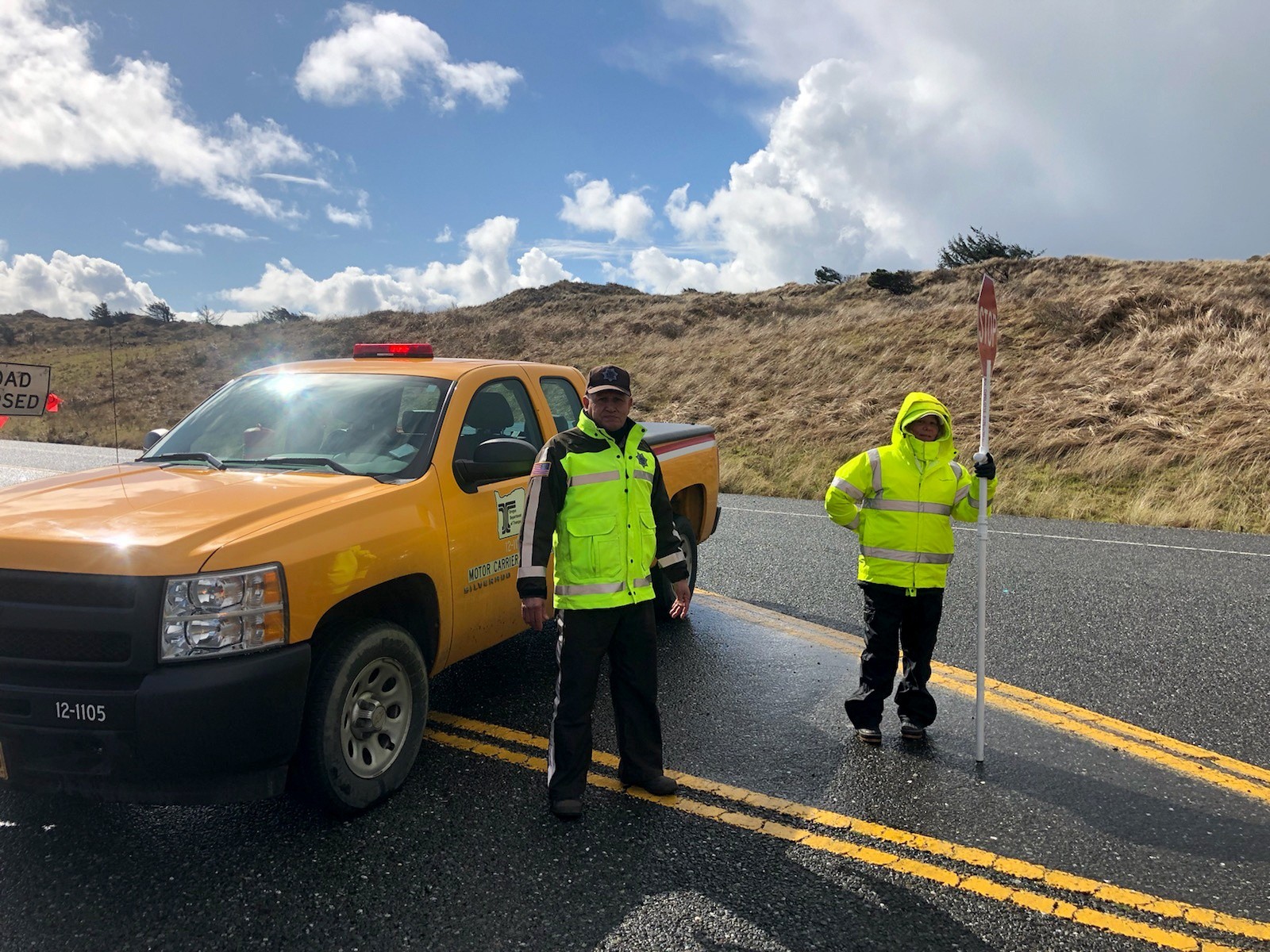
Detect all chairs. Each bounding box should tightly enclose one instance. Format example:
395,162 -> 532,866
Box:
392,408 -> 436,466
454,392 -> 514,460
525,414 -> 569,452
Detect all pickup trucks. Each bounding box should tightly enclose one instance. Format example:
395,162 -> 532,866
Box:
0,341 -> 725,836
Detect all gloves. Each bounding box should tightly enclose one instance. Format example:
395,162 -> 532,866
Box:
974,453 -> 996,480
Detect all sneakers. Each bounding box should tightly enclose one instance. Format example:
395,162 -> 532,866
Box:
548,792 -> 580,816
618,764 -> 678,794
900,714 -> 925,739
856,724 -> 882,743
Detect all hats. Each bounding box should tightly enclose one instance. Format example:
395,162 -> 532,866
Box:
584,365 -> 632,398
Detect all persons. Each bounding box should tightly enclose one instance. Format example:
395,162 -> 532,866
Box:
516,364 -> 690,816
824,393 -> 997,744
